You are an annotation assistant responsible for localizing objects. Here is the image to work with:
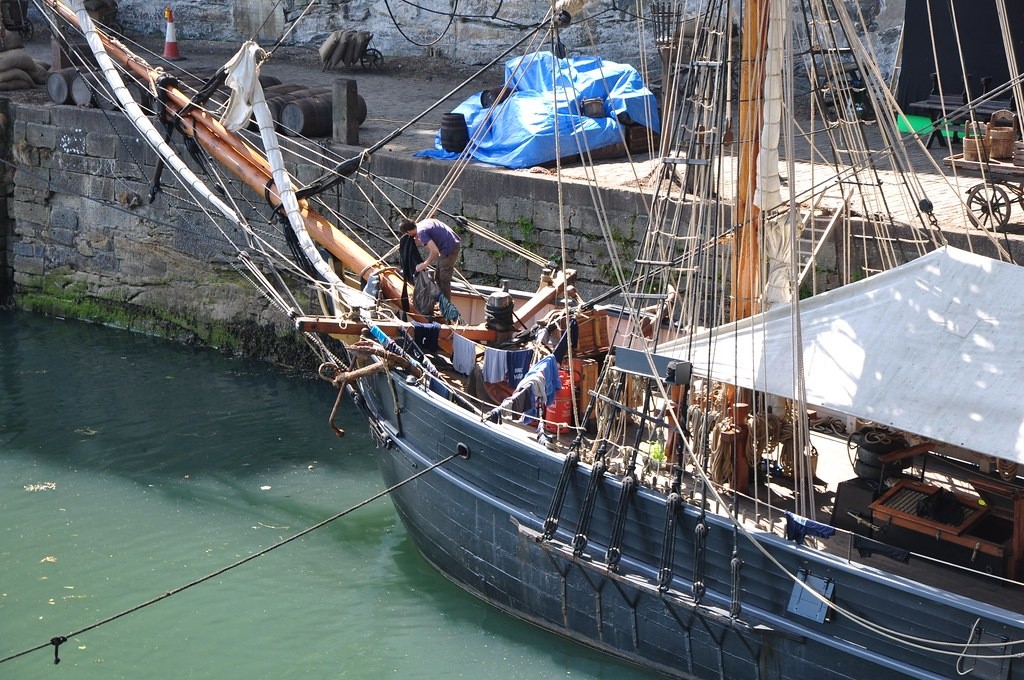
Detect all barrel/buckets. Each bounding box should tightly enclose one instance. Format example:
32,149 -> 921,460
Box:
481,86 -> 517,109
580,96 -> 604,118
962,120 -> 990,160
46,66 -> 367,136
990,109 -> 1017,158
442,113 -> 468,152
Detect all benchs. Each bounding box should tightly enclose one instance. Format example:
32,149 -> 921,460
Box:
867,476 -> 1024,581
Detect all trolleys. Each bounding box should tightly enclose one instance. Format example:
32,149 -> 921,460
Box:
323,33 -> 383,70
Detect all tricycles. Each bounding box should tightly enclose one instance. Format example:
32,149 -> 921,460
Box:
942,150 -> 1024,231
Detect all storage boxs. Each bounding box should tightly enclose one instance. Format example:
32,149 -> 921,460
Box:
562,312 -> 645,425
549,111 -> 662,164
828,473 -> 920,538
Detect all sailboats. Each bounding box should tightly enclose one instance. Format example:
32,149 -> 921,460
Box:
34,0 -> 1024,679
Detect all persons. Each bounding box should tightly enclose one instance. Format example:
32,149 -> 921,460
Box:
399,218 -> 461,314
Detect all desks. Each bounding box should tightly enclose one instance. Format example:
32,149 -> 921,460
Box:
804,63 -> 872,119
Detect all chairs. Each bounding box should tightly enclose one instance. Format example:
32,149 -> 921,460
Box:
909,73 -> 1019,149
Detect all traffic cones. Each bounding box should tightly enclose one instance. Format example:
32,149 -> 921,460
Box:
161,11 -> 181,61
